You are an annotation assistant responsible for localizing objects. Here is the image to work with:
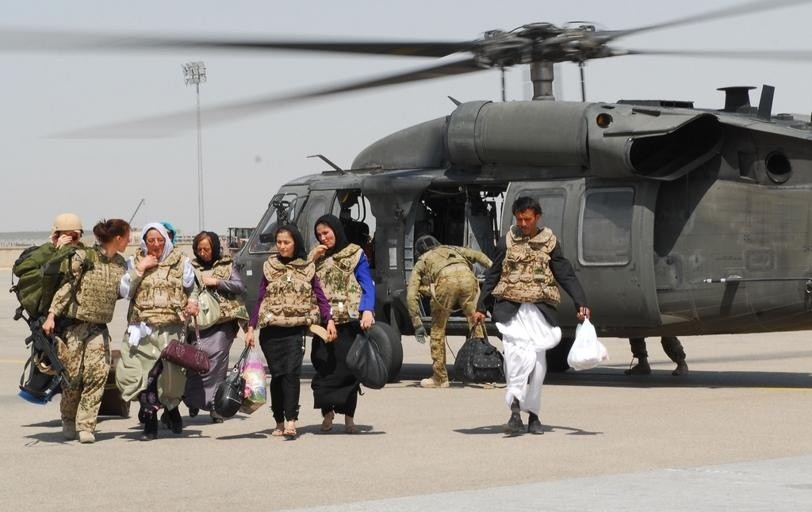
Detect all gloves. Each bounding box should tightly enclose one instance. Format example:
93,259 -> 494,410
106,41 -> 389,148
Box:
414,324 -> 428,344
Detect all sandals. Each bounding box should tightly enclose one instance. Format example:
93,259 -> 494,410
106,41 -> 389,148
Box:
320,412 -> 335,432
209,405 -> 225,424
271,425 -> 299,437
344,424 -> 358,435
189,407 -> 200,418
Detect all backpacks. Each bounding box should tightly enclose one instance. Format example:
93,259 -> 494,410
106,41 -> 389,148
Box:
10,239 -> 96,323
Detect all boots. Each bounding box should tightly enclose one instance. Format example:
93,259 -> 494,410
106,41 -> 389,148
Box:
138,408 -> 158,441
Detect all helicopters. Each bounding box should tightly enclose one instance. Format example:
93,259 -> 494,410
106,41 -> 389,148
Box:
4,1 -> 812,388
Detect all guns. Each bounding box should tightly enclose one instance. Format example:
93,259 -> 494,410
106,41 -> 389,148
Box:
25,315 -> 72,389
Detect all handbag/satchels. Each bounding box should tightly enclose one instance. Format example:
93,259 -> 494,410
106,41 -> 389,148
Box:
214,341 -> 268,419
186,262 -> 221,332
344,328 -> 388,390
451,317 -> 506,384
162,313 -> 211,373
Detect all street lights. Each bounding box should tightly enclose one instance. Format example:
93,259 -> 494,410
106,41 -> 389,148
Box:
182,56 -> 209,233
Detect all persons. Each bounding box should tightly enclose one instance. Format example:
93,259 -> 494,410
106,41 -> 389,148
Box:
245,224 -> 339,437
160,220 -> 176,246
306,212 -> 376,434
41,219 -> 130,444
405,235 -> 493,388
470,196 -> 590,436
118,222 -> 199,441
49,213 -> 84,250
185,230 -> 245,423
623,335 -> 688,376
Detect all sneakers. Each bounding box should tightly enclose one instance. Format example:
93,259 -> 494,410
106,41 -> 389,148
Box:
160,406 -> 184,435
502,413 -> 527,435
60,419 -> 77,440
79,430 -> 96,444
527,414 -> 545,435
420,377 -> 450,389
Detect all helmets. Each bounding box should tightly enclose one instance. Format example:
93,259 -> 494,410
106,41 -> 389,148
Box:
50,212 -> 84,232
414,235 -> 442,260
